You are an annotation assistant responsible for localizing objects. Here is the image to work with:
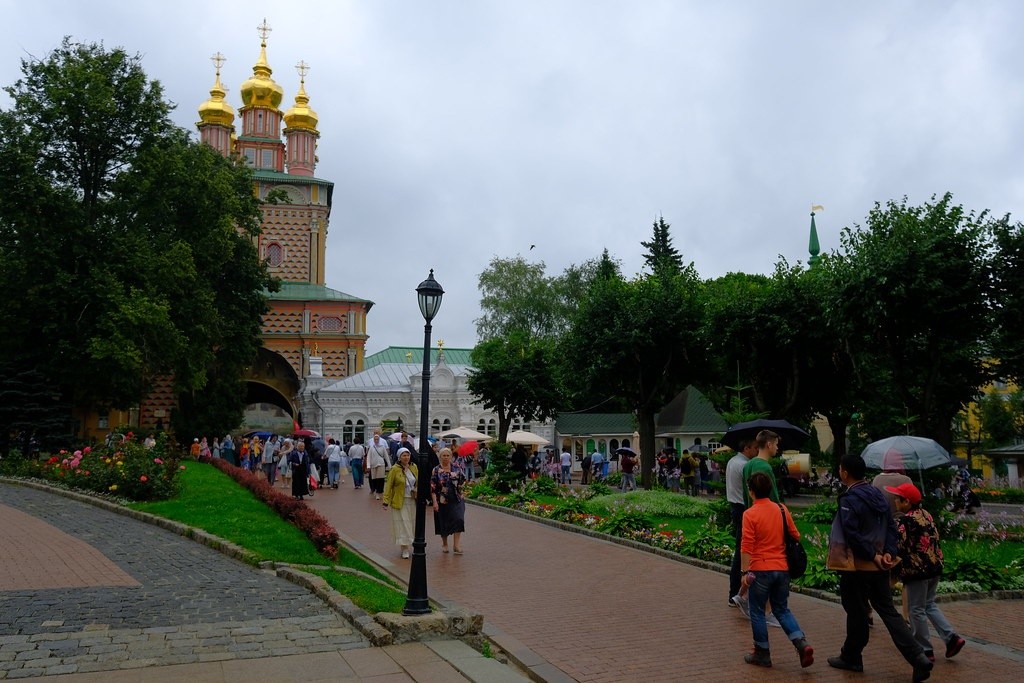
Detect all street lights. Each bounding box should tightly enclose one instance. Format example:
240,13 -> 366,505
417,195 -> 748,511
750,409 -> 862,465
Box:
401,269 -> 445,615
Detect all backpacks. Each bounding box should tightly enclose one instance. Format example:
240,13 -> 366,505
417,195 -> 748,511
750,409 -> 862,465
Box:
680,456 -> 692,475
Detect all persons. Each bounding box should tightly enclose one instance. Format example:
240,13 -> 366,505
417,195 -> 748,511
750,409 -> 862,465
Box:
935,467 -> 981,514
430,448 -> 466,555
826,452 -> 965,683
740,472 -> 814,668
191,429 -> 605,499
656,450 -> 721,496
144,433 -> 156,446
620,455 -> 638,493
380,447 -> 418,558
725,429 -> 782,627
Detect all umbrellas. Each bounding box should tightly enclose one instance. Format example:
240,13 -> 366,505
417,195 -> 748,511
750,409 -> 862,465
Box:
307,430 -> 321,438
388,432 -> 414,446
434,426 -> 492,440
616,447 -> 636,457
861,435 -> 951,470
714,446 -> 732,453
738,572 -> 756,597
292,431 -> 316,436
252,431 -> 272,439
457,441 -> 477,456
503,430 -> 550,444
688,444 -> 710,452
657,447 -> 678,456
719,420 -> 811,454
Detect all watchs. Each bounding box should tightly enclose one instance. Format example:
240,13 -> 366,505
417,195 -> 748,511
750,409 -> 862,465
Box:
740,570 -> 749,575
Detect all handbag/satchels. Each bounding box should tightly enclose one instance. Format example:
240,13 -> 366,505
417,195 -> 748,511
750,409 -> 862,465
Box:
477,457 -> 485,464
775,501 -> 808,580
382,458 -> 388,468
463,453 -> 474,464
309,474 -> 317,490
411,490 -> 417,501
285,462 -> 293,479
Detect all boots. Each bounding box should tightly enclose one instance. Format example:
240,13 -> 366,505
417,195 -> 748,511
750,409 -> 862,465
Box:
795,639 -> 814,668
743,643 -> 772,668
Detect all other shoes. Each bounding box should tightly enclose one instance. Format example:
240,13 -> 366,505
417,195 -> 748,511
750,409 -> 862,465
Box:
441,545 -> 449,553
453,548 -> 463,554
271,483 -> 314,500
400,547 -> 410,559
727,594 -> 736,607
568,478 -> 571,484
331,477 -> 379,501
906,622 -> 911,630
869,617 -> 874,628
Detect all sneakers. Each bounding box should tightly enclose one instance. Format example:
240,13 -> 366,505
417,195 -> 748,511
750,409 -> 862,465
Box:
827,655 -> 863,672
912,652 -> 933,683
925,650 -> 935,662
764,611 -> 781,628
731,595 -> 751,620
945,635 -> 965,658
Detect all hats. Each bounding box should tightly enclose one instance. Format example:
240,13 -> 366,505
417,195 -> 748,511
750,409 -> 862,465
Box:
397,447 -> 410,459
883,483 -> 923,505
194,438 -> 198,441
